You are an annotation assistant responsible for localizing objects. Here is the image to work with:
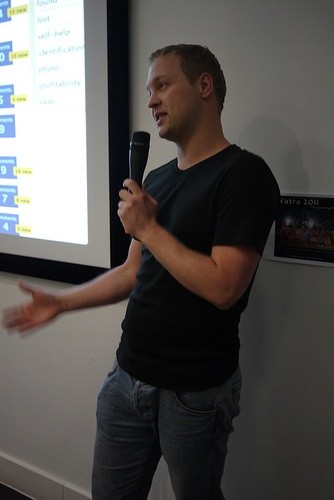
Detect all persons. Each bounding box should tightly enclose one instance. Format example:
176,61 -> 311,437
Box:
0,48 -> 285,500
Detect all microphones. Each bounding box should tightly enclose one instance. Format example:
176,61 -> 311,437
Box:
128,130 -> 150,236
